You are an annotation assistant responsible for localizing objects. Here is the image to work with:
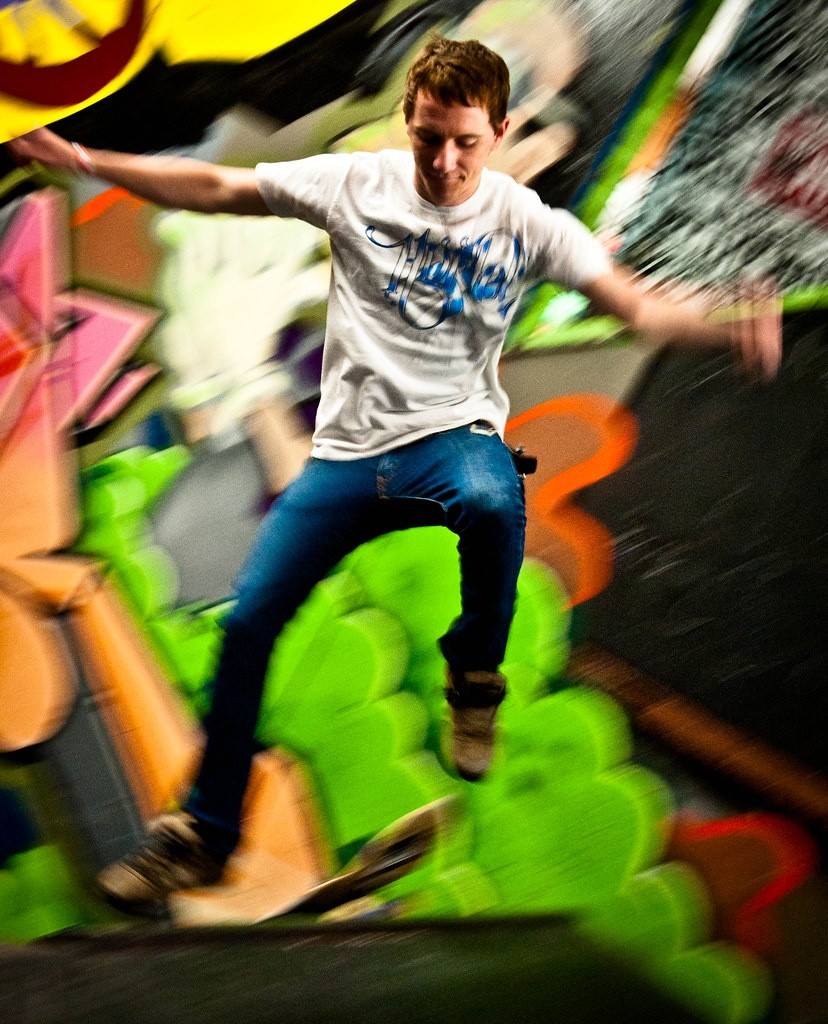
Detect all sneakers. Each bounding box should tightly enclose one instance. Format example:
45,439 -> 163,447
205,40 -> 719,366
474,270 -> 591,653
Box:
84,807 -> 223,917
443,660 -> 509,780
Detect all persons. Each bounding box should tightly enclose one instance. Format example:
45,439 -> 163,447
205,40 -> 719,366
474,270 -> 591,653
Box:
0,40 -> 782,906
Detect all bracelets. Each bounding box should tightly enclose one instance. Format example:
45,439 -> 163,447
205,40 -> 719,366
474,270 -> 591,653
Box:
67,138 -> 97,185
